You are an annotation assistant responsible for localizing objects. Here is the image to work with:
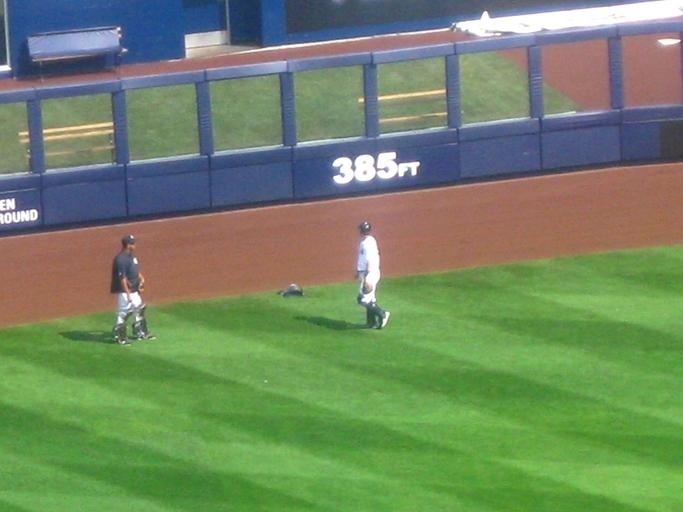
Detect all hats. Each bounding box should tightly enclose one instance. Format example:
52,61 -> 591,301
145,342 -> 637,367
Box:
122,234 -> 138,245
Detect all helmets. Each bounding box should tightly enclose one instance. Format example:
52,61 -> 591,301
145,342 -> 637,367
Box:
286,282 -> 305,296
357,221 -> 371,236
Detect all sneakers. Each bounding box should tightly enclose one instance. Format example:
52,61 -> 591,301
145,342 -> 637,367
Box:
360,320 -> 378,329
375,311 -> 391,329
137,333 -> 158,341
116,338 -> 132,347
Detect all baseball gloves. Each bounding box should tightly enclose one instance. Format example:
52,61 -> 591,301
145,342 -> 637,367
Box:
363,283 -> 373,294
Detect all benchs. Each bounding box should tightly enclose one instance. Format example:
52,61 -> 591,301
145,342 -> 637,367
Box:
26,26 -> 125,82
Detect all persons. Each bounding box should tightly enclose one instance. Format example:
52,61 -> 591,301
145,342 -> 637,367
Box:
108,234 -> 156,345
353,221 -> 392,329
